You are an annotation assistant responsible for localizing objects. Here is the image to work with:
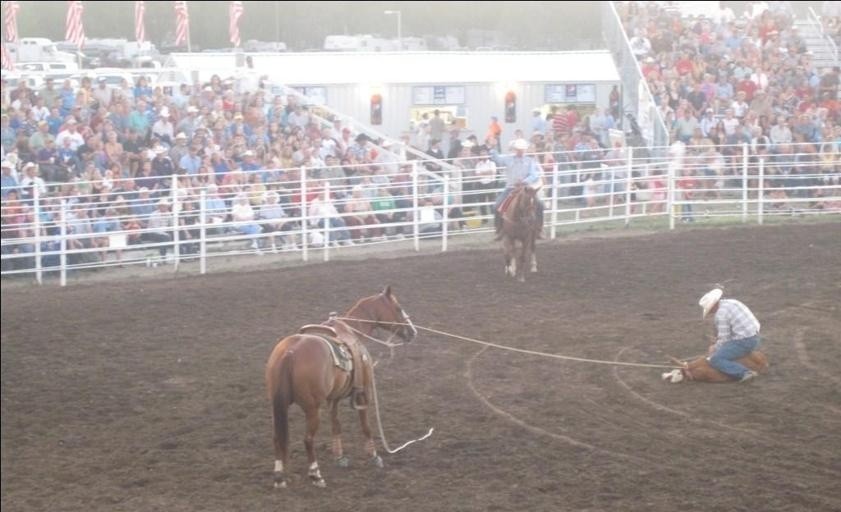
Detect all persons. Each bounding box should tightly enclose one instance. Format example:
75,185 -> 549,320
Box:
1,1 -> 841,280
699,288 -> 763,383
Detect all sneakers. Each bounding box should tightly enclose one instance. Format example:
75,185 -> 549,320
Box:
493,228 -> 504,241
739,370 -> 759,384
537,231 -> 545,241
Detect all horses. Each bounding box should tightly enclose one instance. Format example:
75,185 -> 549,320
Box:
503,186 -> 541,282
265,285 -> 416,490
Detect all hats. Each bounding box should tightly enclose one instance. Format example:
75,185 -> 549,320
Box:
699,288 -> 723,320
509,138 -> 531,151
